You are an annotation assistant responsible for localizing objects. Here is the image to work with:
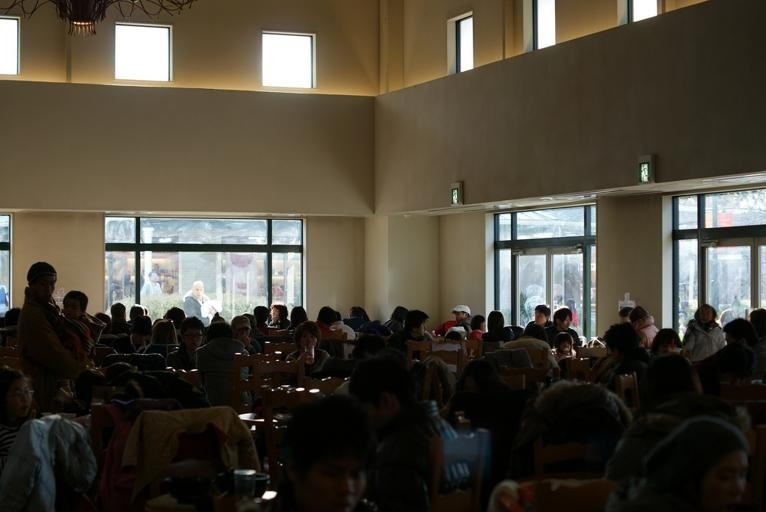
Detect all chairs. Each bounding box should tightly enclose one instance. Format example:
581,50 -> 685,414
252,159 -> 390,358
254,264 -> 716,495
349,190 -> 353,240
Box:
0,327 -> 766,512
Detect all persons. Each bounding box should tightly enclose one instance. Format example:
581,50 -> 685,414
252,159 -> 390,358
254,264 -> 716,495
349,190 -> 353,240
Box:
1,260 -> 766,512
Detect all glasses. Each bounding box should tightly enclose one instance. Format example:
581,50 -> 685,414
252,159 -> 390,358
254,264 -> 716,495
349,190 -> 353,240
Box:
10,390 -> 34,399
183,332 -> 202,338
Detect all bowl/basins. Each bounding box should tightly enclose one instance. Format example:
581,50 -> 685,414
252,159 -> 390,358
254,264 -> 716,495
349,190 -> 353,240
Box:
235,472 -> 270,499
162,476 -> 224,502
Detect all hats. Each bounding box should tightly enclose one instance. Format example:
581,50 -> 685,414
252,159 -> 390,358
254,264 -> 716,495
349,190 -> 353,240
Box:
232,316 -> 251,330
27,262 -> 56,284
451,304 -> 472,316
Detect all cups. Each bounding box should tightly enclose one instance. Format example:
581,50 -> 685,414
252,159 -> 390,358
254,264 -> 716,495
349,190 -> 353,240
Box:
234,470 -> 257,510
304,343 -> 315,360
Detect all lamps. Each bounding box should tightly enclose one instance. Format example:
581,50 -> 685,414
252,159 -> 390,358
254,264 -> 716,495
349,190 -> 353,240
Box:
0,0 -> 196,38
